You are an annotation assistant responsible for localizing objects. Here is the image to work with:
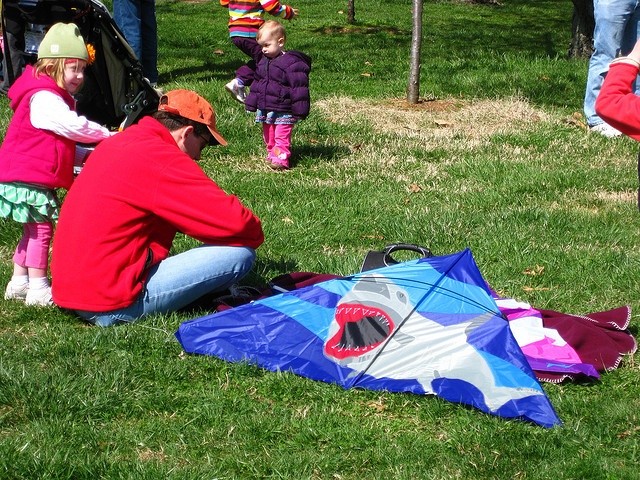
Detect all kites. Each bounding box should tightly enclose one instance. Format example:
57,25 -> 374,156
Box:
174,247 -> 564,428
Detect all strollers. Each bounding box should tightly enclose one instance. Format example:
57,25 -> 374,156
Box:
0,1 -> 162,131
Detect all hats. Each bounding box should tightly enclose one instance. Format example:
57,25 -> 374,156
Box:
158,90 -> 227,146
38,23 -> 95,64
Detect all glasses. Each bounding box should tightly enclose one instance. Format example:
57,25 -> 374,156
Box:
193,130 -> 210,149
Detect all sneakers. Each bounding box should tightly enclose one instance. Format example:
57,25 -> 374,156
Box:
589,124 -> 622,138
265,155 -> 285,168
25,288 -> 55,308
4,279 -> 28,300
225,80 -> 246,104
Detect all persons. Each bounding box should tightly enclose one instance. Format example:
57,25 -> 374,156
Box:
50,90 -> 264,328
595,38 -> 640,141
0,22 -> 121,308
245,20 -> 311,170
113,0 -> 163,97
584,0 -> 640,137
220,0 -> 300,104
